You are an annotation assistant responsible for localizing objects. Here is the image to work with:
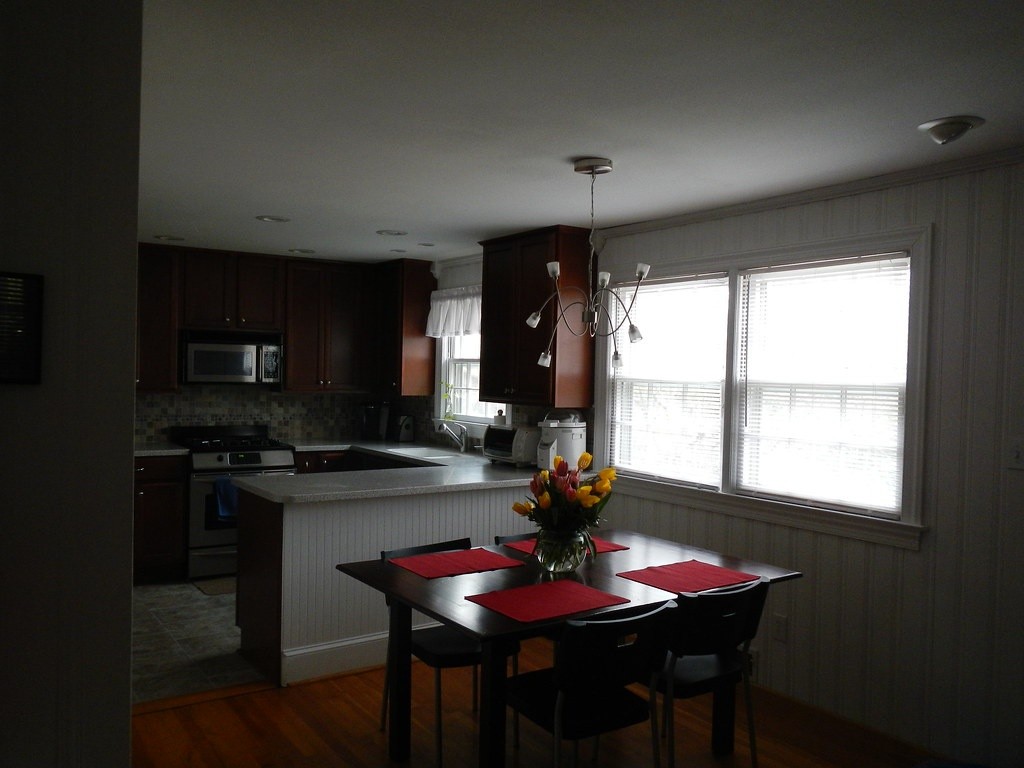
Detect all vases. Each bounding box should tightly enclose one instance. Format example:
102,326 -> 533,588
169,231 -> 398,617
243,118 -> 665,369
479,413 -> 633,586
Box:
537,530 -> 587,572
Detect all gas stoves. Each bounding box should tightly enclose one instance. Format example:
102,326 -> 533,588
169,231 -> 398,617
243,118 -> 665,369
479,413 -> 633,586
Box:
167,424 -> 297,472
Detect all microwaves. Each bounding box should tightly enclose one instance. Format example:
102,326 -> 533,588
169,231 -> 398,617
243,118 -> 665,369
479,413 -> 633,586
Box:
178,326 -> 284,386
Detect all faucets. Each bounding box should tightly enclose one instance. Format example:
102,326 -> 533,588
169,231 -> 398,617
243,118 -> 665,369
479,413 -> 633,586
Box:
437,422 -> 469,452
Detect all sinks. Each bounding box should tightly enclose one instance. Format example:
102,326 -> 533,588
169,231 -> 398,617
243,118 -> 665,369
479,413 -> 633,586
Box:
387,448 -> 462,459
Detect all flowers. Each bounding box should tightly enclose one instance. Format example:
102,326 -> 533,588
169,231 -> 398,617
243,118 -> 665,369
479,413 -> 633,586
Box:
507,445 -> 620,535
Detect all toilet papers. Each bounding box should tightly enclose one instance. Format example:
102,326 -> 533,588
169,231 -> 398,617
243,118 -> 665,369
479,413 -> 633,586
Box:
494,415 -> 506,424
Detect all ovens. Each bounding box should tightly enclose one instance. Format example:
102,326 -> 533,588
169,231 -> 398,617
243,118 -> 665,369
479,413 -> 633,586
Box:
182,470 -> 295,579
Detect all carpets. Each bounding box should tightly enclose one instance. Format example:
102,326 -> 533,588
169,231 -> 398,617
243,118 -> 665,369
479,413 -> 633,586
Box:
188,574 -> 237,596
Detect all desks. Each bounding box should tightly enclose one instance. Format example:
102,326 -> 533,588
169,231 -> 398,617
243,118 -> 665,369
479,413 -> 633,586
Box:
335,527 -> 803,741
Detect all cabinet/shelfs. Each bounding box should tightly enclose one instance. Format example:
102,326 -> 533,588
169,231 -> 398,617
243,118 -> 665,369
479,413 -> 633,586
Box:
477,224 -> 597,409
132,455 -> 191,587
135,241 -> 438,398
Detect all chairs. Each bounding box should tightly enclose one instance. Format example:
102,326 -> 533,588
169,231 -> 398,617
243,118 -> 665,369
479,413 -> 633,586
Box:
380,529 -> 769,768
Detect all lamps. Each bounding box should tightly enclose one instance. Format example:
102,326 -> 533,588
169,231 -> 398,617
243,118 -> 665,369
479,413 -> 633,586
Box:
915,115 -> 985,147
526,158 -> 651,368
